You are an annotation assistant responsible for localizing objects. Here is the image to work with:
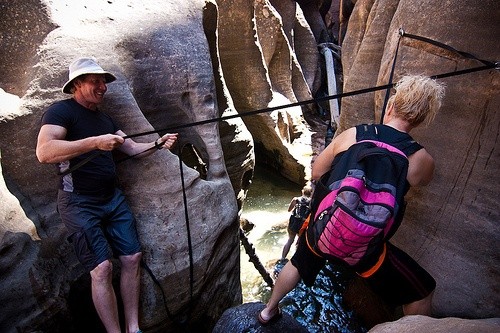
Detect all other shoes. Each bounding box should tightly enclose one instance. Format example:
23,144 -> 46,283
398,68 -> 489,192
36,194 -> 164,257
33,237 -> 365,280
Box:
278,259 -> 288,265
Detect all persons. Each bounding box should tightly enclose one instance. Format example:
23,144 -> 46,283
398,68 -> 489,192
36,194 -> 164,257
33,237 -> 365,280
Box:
257,73 -> 446,326
36,57 -> 178,332
281,185 -> 312,258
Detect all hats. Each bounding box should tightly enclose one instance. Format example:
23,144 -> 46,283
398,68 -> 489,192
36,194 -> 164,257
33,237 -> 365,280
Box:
62,58 -> 117,94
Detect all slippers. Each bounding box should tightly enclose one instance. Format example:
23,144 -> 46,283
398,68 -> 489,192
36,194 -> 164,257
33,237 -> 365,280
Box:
258,307 -> 282,325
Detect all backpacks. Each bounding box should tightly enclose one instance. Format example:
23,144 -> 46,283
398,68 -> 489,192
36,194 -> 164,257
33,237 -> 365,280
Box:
289,197 -> 311,233
307,124 -> 424,266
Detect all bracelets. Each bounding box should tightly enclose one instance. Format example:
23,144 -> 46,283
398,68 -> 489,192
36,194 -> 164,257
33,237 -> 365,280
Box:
155,138 -> 163,150
